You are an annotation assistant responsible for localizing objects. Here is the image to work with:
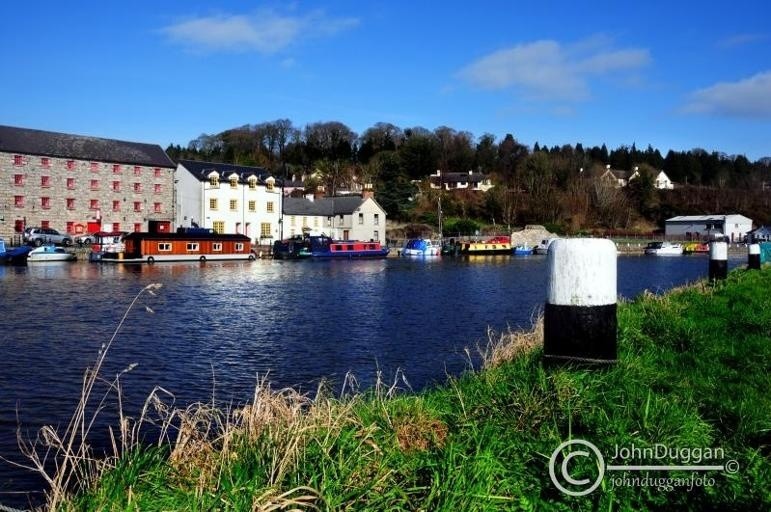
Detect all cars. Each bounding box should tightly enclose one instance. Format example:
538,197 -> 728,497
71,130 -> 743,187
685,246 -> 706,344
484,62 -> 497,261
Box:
75,232 -> 121,245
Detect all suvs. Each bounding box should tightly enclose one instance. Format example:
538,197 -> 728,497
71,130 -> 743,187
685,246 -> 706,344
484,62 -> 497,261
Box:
23,227 -> 72,247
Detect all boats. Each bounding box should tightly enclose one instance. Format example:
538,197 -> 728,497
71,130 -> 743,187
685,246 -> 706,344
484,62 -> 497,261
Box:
89,221 -> 256,262
401,236 -> 554,257
0,244 -> 76,263
274,236 -> 391,261
644,241 -> 709,259
